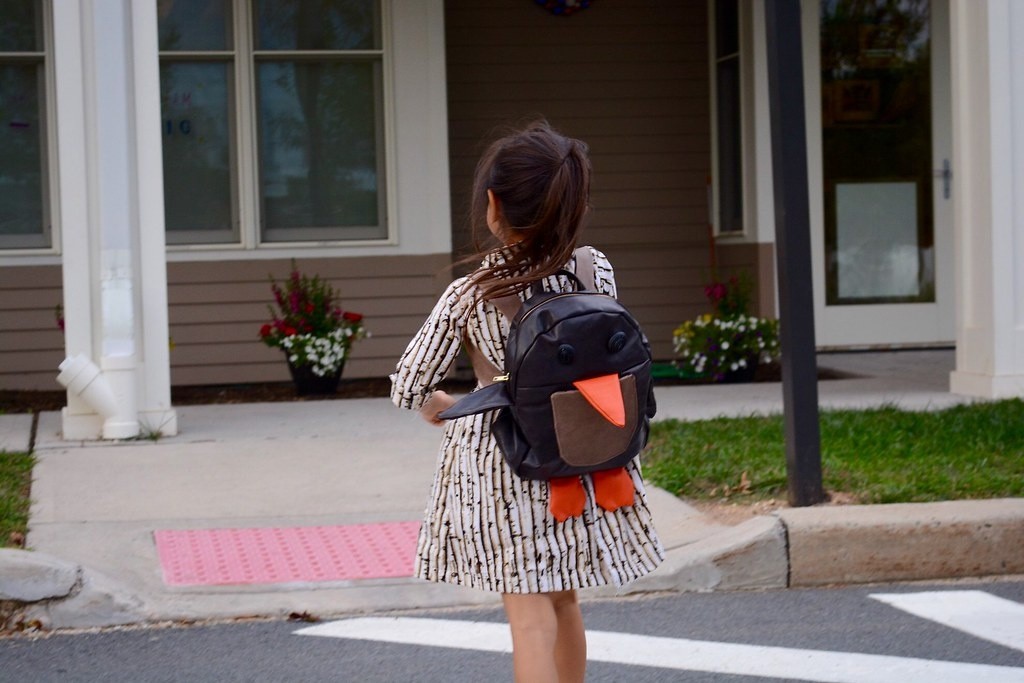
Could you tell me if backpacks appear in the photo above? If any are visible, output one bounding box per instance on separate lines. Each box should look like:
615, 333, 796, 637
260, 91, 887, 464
433, 247, 659, 520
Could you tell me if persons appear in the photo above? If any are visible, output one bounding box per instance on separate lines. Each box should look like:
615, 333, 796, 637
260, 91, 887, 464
387, 117, 665, 683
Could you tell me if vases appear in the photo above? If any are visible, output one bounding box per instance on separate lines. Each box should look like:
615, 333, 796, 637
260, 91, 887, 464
286, 353, 345, 398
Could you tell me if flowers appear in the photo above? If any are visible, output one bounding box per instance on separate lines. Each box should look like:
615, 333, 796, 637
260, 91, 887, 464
669, 273, 781, 385
255, 256, 372, 375
